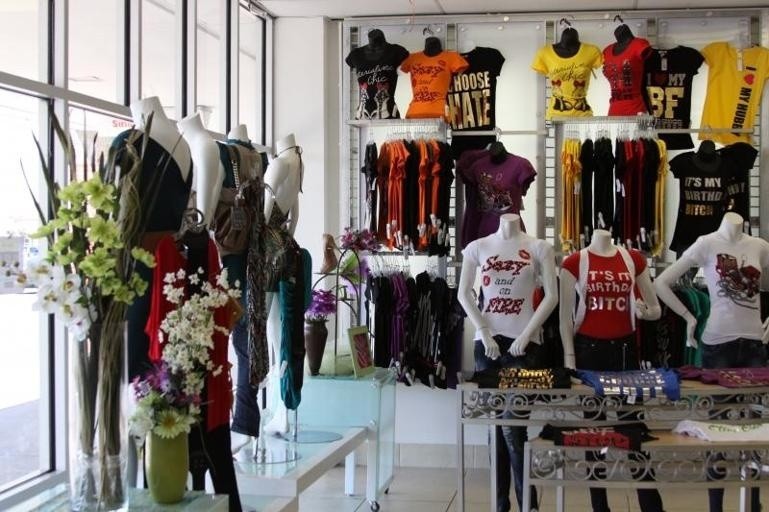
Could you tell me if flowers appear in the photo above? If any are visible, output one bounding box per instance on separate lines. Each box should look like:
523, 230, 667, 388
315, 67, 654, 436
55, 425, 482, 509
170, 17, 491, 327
304, 289, 337, 322
20, 105, 184, 512
128, 266, 242, 460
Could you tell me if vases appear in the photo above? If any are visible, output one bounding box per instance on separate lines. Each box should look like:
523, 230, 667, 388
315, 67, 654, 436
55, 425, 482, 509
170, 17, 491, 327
145, 431, 188, 504
304, 320, 330, 376
71, 450, 129, 512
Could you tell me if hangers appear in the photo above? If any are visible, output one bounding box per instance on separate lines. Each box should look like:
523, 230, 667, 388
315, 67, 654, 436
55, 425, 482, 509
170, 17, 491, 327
369, 250, 448, 282
366, 116, 448, 145
563, 114, 659, 143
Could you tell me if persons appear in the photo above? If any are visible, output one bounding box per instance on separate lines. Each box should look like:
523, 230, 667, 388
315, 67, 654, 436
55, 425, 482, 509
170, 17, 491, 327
458, 213, 559, 511
212, 124, 264, 438
174, 114, 218, 235
651, 212, 767, 511
561, 229, 663, 511
258, 134, 303, 440
108, 98, 192, 488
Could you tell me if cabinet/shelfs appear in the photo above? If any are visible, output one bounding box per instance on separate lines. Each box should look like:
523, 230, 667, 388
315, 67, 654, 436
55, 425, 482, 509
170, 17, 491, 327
456, 369, 769, 512
55, 365, 397, 512
347, 6, 763, 327
